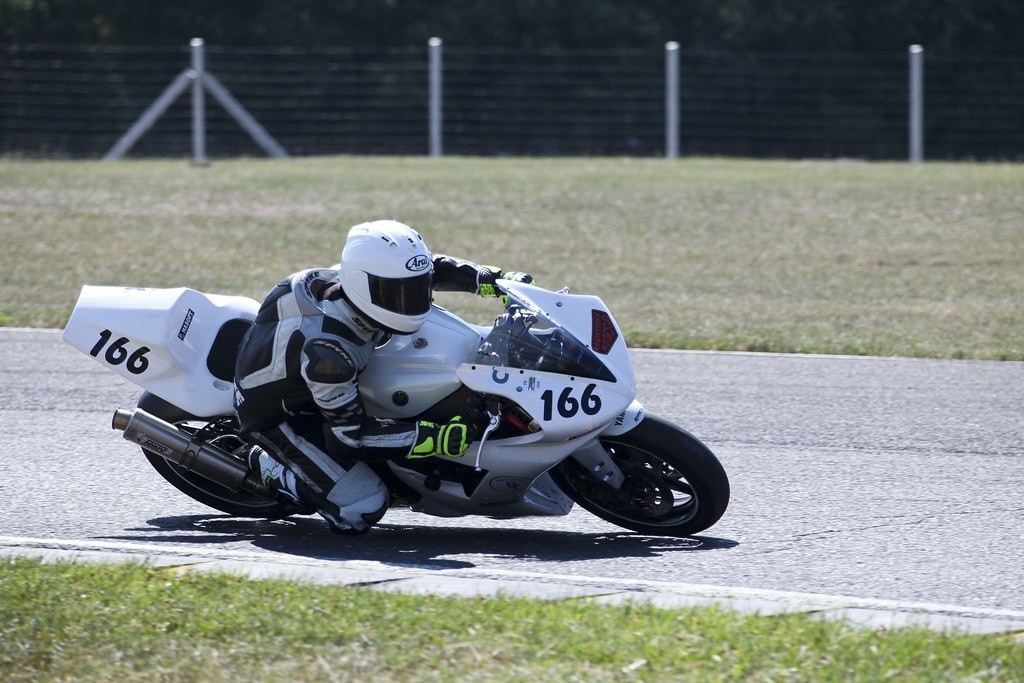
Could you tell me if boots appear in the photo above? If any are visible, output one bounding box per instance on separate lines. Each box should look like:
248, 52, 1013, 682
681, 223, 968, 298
249, 444, 303, 505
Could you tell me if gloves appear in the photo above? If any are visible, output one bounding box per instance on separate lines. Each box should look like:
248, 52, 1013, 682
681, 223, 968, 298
407, 415, 477, 457
479, 265, 535, 308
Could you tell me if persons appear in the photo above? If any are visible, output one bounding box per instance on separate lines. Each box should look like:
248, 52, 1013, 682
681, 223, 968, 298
232, 219, 537, 535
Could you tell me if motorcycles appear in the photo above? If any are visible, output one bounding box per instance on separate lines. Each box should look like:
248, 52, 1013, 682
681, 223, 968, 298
58, 263, 731, 538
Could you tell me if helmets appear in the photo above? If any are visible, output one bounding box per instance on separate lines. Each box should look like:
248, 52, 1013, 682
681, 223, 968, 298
340, 220, 434, 336
